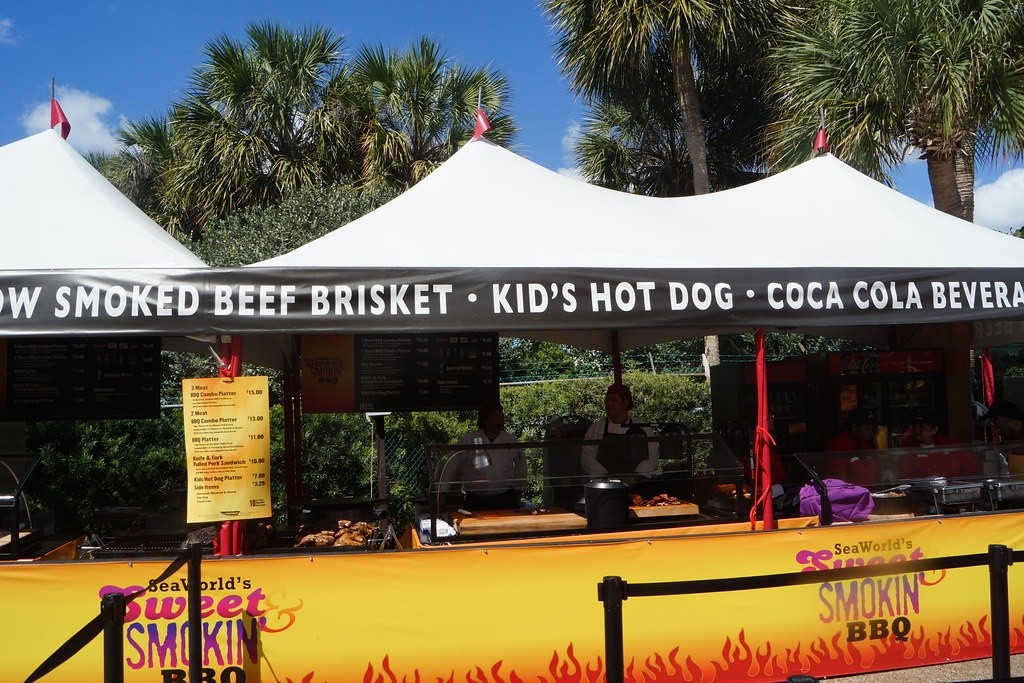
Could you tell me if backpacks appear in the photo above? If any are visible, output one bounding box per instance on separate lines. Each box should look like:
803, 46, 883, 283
799, 479, 876, 522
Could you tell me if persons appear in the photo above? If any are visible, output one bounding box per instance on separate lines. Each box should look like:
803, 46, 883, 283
586, 384, 659, 481
435, 402, 527, 511
898, 406, 980, 479
825, 407, 883, 482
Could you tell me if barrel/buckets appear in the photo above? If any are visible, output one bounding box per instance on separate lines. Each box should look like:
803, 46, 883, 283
584, 478, 630, 532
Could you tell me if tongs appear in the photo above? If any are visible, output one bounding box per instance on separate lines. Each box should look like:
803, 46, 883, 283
873, 484, 912, 493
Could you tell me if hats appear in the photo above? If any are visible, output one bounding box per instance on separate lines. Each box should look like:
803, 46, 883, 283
608, 383, 633, 409
477, 401, 503, 428
904, 412, 936, 425
854, 412, 886, 426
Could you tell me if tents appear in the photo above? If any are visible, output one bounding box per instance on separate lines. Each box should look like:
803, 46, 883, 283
0, 129, 1024, 559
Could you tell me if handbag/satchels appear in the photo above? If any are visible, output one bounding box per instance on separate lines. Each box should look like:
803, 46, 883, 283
773, 485, 794, 516
